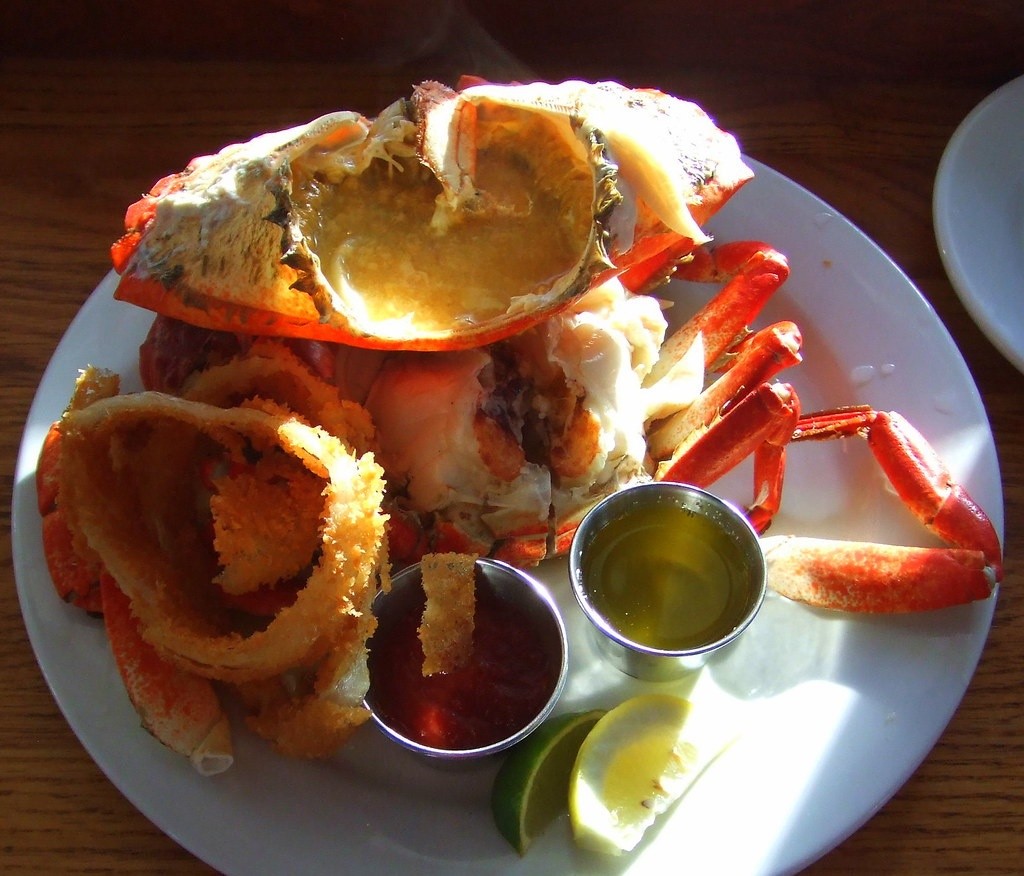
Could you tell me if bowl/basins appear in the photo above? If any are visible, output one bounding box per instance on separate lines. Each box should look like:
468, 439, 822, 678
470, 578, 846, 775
355, 556, 568, 775
567, 481, 769, 683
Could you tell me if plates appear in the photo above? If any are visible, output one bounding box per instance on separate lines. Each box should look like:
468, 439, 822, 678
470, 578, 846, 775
931, 74, 1024, 378
10, 143, 1001, 876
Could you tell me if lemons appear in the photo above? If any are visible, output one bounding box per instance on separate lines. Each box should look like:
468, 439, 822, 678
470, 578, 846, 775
492, 708, 609, 855
570, 694, 751, 855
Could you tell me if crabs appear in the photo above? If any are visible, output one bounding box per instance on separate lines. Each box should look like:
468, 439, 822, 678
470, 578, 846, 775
34, 70, 1005, 780
